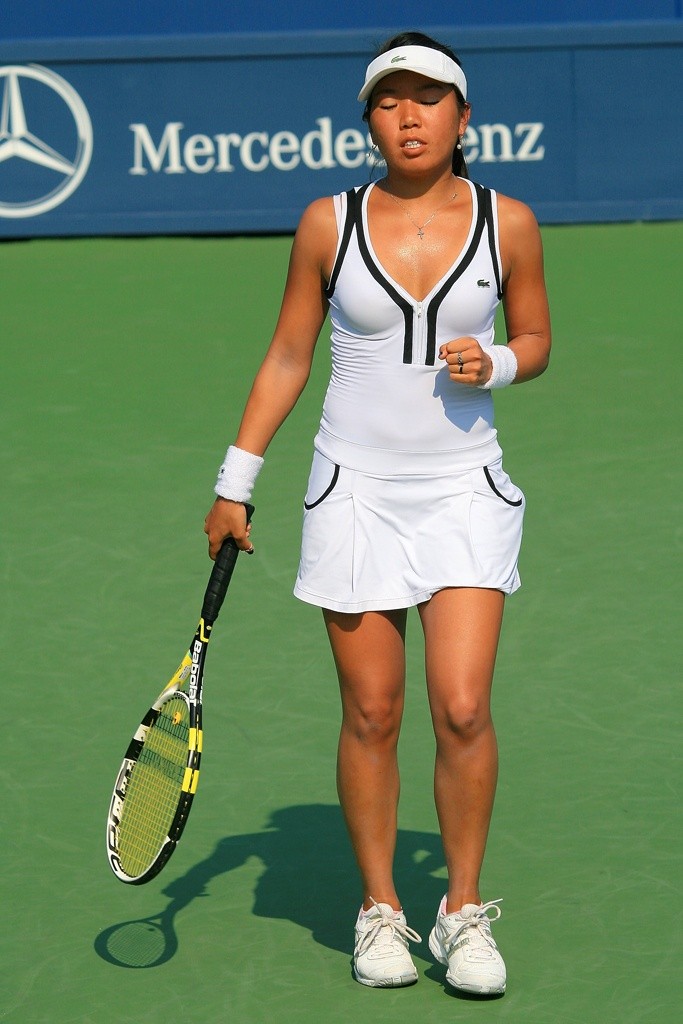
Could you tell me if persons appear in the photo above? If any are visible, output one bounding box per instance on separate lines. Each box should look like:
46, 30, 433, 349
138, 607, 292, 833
204, 32, 550, 993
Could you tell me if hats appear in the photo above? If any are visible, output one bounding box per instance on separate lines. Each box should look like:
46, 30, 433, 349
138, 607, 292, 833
357, 45, 467, 103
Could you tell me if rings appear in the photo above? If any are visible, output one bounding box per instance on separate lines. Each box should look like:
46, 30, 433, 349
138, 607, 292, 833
458, 352, 463, 373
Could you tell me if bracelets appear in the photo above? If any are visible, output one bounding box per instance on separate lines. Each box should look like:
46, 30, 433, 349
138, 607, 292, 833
477, 344, 517, 391
214, 445, 264, 502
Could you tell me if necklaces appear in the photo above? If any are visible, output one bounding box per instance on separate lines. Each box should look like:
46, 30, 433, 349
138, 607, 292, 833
382, 177, 456, 240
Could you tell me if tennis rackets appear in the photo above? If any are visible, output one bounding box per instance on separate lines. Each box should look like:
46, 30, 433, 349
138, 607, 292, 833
104, 502, 257, 886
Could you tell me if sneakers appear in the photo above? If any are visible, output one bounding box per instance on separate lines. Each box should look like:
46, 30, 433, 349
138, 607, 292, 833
353, 895, 419, 989
428, 892, 507, 995
